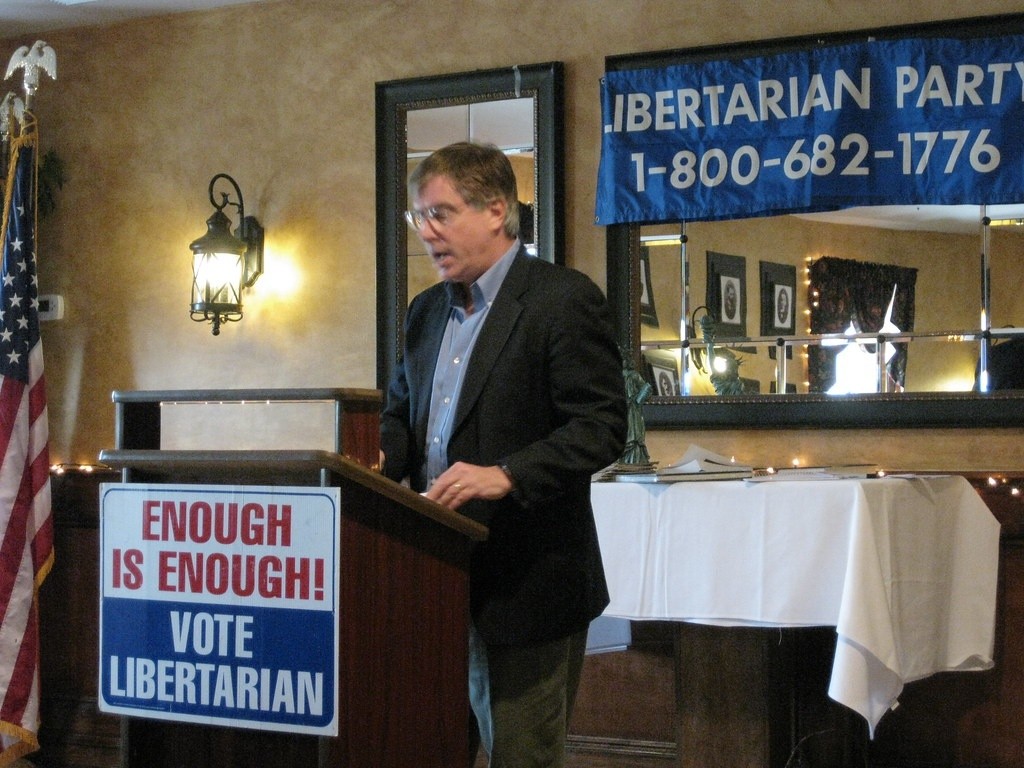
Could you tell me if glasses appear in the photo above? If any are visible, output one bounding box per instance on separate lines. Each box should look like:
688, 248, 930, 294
404, 193, 480, 233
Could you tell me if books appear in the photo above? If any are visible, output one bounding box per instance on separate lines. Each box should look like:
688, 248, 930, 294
777, 463, 878, 474
615, 470, 753, 483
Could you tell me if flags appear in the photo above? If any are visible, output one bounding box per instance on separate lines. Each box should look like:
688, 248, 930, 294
0, 110, 54, 768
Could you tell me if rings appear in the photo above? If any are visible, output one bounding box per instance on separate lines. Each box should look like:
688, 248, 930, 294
453, 483, 462, 490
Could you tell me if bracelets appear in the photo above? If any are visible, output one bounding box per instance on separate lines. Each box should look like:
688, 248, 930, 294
495, 459, 513, 482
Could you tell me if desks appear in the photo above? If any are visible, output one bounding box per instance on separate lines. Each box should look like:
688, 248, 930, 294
591, 475, 1024, 768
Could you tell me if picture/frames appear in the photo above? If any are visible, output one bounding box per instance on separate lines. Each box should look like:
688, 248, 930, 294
758, 260, 800, 336
704, 250, 749, 337
637, 247, 663, 327
643, 349, 678, 396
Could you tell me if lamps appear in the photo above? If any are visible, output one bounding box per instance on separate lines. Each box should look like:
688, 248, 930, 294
684, 307, 741, 374
185, 169, 266, 337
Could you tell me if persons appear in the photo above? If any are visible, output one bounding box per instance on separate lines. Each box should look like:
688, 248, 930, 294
379, 140, 629, 768
973, 338, 1024, 392
621, 357, 651, 464
701, 328, 746, 396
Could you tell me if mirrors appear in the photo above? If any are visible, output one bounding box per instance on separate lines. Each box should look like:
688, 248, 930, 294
603, 9, 1024, 430
376, 60, 564, 390
